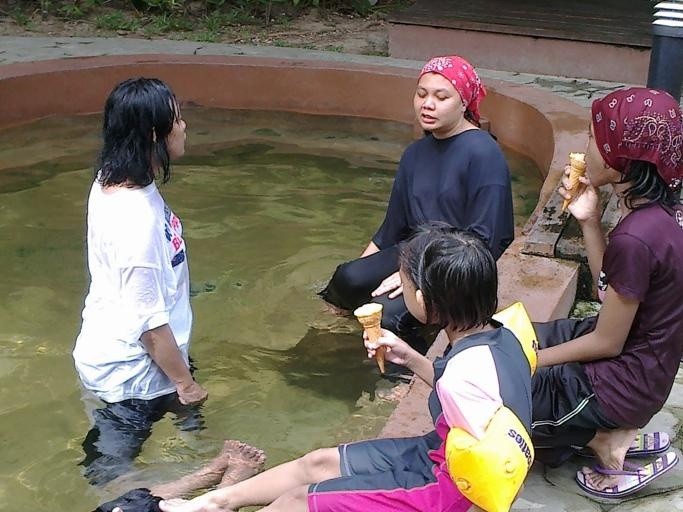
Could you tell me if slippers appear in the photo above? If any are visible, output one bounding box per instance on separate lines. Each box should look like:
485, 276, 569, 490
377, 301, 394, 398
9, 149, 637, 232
575, 452, 679, 499
573, 430, 671, 457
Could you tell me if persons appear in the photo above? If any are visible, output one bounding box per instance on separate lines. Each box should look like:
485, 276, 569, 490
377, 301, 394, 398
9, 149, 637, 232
157, 220, 533, 512
317, 53, 513, 402
509, 85, 683, 499
91, 439, 267, 512
71, 76, 210, 489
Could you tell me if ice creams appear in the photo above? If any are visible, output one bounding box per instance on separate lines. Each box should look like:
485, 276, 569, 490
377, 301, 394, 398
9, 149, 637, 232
354, 301, 386, 375
561, 151, 587, 212
